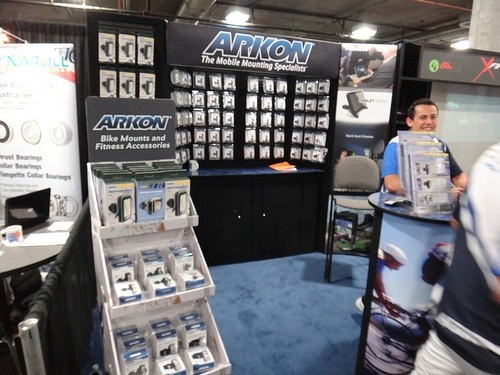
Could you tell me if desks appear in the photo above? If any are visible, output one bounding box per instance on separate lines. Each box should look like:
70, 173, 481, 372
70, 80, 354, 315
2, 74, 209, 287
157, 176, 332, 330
356, 190, 456, 375
0, 216, 75, 349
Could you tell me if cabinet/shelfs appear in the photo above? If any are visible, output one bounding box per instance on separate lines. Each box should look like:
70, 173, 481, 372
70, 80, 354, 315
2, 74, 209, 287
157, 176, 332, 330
193, 178, 321, 267
86, 159, 232, 375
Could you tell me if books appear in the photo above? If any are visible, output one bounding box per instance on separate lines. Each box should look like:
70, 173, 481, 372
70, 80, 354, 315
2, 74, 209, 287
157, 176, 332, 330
269, 161, 297, 171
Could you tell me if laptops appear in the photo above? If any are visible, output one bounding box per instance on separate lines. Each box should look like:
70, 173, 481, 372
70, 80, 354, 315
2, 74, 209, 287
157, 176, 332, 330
0, 187, 51, 236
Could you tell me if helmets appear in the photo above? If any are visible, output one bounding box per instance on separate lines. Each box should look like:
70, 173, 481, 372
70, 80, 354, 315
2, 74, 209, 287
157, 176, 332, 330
383, 243, 405, 268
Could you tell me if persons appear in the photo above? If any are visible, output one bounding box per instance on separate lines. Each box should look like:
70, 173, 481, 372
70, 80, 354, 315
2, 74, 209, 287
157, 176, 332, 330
450, 204, 461, 229
356, 98, 469, 312
409, 141, 500, 375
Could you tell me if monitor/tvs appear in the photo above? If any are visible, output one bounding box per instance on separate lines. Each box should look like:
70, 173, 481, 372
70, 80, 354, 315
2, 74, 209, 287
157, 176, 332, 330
347, 90, 367, 113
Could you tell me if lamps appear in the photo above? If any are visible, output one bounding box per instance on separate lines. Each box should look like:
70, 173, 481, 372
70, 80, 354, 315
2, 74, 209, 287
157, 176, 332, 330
225, 5, 250, 22
350, 21, 378, 40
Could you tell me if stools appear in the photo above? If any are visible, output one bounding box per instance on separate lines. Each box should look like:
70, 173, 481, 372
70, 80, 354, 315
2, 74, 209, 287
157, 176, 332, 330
324, 155, 381, 284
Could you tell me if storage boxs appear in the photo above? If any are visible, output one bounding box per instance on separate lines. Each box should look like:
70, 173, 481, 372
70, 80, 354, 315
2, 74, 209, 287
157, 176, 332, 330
105, 245, 206, 306
90, 161, 191, 224
114, 311, 215, 375
